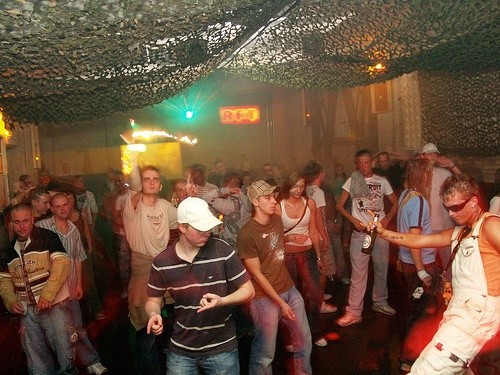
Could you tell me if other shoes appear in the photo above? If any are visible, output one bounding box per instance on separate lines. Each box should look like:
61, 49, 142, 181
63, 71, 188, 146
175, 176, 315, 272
320, 302, 337, 312
316, 338, 326, 347
341, 276, 350, 285
96, 312, 106, 320
323, 292, 331, 301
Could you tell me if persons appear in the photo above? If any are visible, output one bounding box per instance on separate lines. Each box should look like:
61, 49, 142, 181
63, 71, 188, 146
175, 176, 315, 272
0, 143, 500, 375
359, 173, 500, 375
145, 197, 256, 375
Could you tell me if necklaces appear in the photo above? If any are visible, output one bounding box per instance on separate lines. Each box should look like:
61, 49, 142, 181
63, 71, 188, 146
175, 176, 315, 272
286, 198, 302, 206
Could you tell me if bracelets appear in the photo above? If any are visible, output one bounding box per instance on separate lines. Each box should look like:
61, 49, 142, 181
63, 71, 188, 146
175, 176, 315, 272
450, 164, 456, 169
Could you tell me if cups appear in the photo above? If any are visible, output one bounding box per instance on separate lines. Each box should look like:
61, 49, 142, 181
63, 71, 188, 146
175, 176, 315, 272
20, 301, 27, 315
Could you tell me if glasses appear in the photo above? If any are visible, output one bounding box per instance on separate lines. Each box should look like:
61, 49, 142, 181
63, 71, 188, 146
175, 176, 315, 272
442, 193, 476, 213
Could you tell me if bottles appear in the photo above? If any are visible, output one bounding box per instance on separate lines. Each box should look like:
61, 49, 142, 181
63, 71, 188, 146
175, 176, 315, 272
361, 214, 379, 255
410, 282, 425, 304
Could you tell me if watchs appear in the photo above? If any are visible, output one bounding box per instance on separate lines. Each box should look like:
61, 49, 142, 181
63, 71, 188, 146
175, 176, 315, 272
317, 257, 321, 261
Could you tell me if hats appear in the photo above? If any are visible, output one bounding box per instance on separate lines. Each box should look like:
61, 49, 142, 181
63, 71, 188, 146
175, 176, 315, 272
246, 180, 278, 202
422, 142, 439, 152
176, 196, 223, 232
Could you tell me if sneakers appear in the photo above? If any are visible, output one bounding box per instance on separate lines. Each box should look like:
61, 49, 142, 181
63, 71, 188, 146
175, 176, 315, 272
371, 300, 396, 316
336, 313, 360, 327
88, 362, 107, 375
